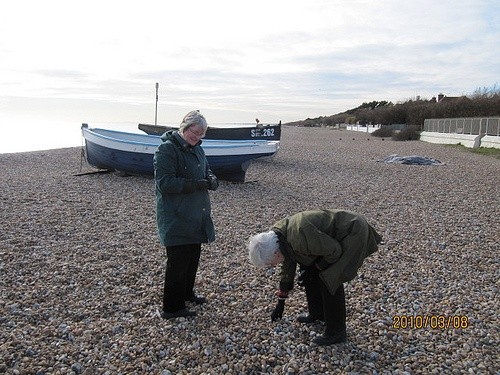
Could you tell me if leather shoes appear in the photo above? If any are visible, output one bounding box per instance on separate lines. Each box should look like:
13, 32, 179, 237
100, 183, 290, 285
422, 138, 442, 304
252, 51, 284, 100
312, 330, 347, 345
160, 307, 197, 320
186, 294, 207, 306
296, 313, 321, 323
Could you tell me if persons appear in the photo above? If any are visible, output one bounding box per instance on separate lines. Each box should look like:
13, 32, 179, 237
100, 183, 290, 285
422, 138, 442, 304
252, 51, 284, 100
155, 110, 217, 318
248, 210, 382, 346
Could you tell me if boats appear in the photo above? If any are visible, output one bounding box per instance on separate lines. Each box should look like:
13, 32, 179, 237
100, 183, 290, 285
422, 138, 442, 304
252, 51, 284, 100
138, 82, 282, 141
81, 122, 281, 182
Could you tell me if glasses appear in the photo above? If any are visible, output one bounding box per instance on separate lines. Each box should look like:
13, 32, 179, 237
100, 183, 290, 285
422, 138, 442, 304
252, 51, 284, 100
188, 127, 205, 138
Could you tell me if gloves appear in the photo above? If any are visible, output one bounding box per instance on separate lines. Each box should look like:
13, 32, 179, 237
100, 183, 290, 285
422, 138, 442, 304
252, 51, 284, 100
192, 178, 209, 191
297, 263, 323, 288
271, 297, 286, 324
207, 174, 219, 191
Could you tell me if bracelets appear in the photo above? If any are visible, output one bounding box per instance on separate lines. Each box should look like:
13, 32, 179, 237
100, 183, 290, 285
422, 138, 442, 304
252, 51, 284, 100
278, 297, 286, 301
